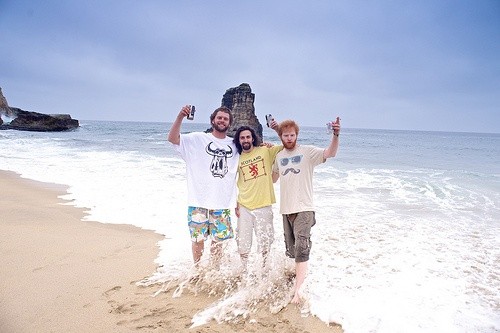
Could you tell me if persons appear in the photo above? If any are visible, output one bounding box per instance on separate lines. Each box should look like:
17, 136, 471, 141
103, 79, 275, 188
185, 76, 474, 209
261, 116, 341, 305
235, 114, 282, 280
168, 105, 275, 278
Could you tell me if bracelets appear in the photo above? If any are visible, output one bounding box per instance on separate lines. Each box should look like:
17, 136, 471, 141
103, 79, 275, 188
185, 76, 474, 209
331, 130, 339, 136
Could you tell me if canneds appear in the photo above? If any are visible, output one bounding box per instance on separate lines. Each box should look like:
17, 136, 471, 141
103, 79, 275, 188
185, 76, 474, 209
187, 105, 195, 120
325, 120, 335, 135
265, 113, 273, 127
